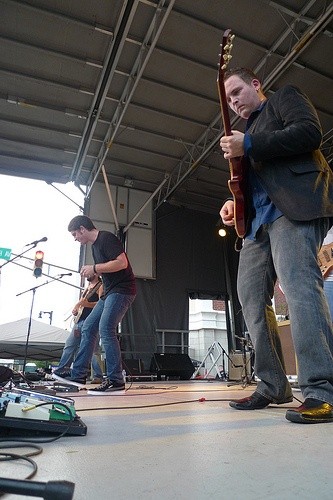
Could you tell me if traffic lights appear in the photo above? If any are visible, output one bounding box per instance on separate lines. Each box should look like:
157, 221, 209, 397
33, 250, 44, 277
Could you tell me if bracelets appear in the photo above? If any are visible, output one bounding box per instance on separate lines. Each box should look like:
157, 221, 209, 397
93, 263, 97, 274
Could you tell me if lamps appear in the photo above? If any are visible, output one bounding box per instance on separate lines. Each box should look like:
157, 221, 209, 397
215, 219, 232, 238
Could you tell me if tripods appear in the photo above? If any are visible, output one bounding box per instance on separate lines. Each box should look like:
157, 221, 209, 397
222, 241, 258, 389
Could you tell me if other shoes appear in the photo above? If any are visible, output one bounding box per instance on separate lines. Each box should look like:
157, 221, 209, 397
61, 379, 86, 387
285, 399, 333, 423
229, 391, 294, 410
92, 378, 103, 384
89, 379, 126, 394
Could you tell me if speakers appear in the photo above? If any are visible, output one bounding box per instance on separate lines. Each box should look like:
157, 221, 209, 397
277, 319, 298, 380
149, 352, 196, 381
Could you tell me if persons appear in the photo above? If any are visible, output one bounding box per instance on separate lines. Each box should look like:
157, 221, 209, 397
53, 216, 138, 393
45, 272, 105, 384
220, 65, 333, 423
322, 226, 333, 325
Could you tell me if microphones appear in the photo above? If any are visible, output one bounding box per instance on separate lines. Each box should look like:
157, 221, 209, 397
26, 237, 47, 246
60, 272, 72, 276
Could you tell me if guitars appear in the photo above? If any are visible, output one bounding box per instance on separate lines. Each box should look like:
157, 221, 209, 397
216, 28, 252, 238
74, 288, 92, 324
278, 241, 333, 301
